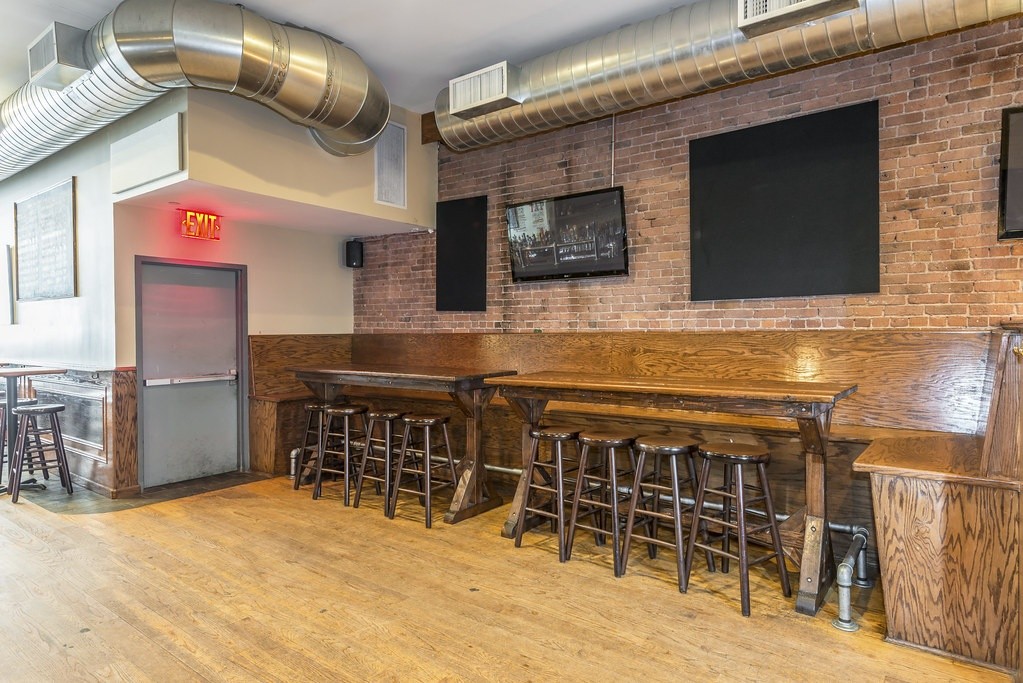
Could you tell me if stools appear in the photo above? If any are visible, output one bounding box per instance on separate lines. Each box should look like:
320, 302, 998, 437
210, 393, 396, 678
515, 425, 791, 616
293, 401, 458, 528
0, 397, 74, 504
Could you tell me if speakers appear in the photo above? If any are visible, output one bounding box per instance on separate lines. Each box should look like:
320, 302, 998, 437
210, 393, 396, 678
346, 241, 364, 268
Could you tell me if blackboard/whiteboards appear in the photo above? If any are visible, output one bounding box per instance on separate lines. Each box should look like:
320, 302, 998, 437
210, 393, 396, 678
689, 100, 880, 301
436, 195, 487, 311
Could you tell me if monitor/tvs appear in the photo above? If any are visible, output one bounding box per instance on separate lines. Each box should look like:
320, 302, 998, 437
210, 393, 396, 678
506, 186, 630, 283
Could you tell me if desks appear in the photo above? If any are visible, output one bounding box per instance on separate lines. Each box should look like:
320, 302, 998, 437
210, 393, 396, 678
285, 363, 518, 524
0, 366, 67, 494
484, 371, 859, 617
